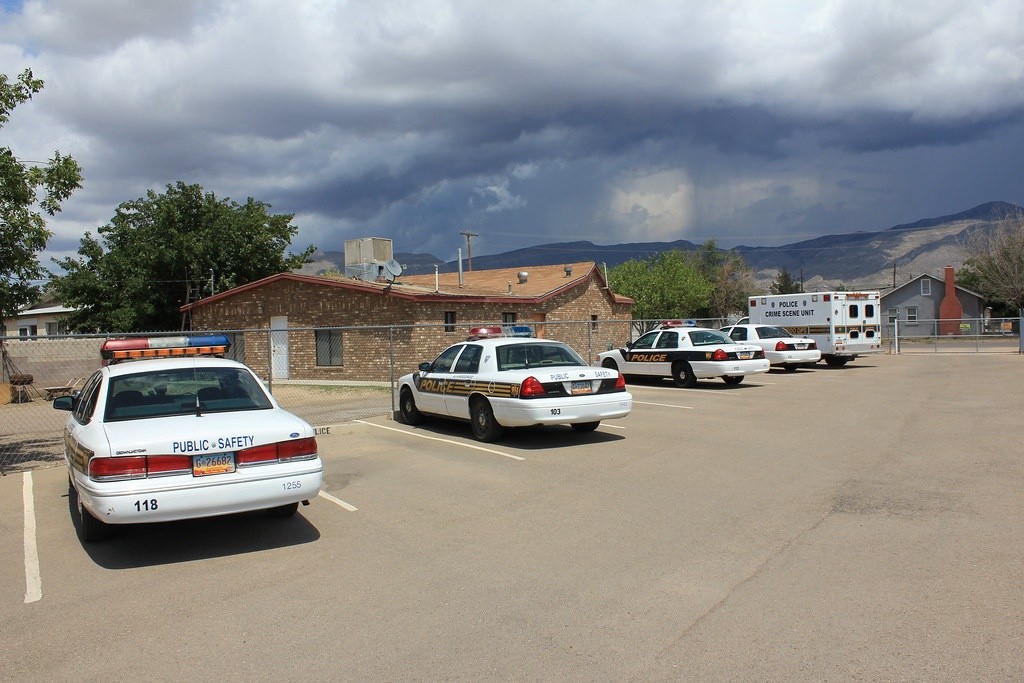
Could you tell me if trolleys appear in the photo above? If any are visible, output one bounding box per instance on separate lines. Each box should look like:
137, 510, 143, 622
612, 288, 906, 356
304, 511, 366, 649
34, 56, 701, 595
44, 377, 86, 401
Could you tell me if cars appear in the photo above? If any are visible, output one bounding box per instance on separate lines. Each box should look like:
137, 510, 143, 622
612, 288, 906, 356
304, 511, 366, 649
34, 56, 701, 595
396, 326, 633, 443
717, 324, 822, 373
53, 334, 325, 544
594, 320, 771, 388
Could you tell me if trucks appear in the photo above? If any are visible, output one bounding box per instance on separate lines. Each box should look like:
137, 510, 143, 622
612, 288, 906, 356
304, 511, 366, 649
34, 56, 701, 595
734, 291, 885, 368
